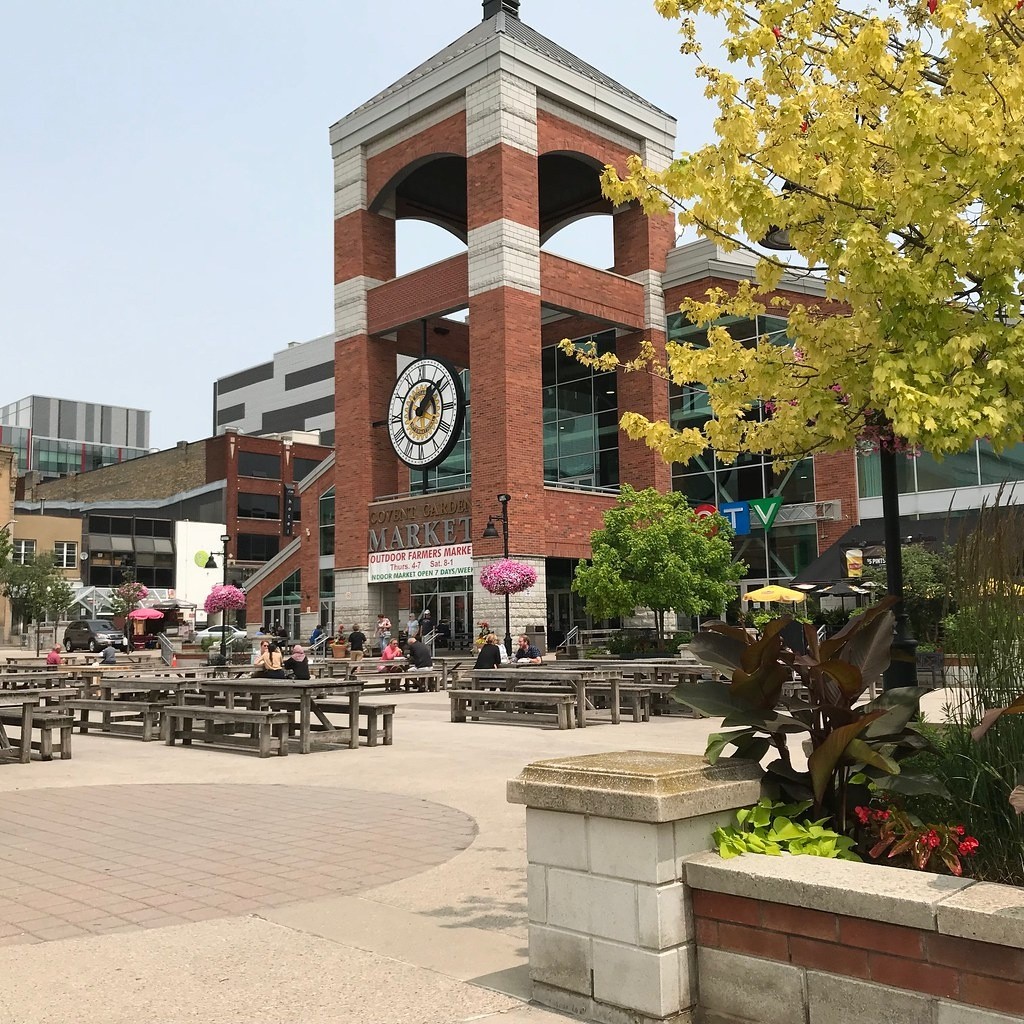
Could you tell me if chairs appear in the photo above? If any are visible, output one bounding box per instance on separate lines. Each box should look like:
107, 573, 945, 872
0, 653, 715, 760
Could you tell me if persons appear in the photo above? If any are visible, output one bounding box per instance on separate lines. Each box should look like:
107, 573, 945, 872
276, 626, 290, 649
284, 644, 310, 680
47, 643, 65, 664
348, 623, 368, 671
436, 619, 450, 648
374, 614, 391, 655
403, 613, 420, 649
253, 640, 270, 662
419, 610, 432, 656
268, 627, 277, 636
494, 635, 508, 664
101, 641, 116, 664
510, 634, 542, 664
407, 637, 435, 693
310, 625, 323, 655
256, 627, 265, 636
473, 633, 501, 669
376, 638, 404, 692
254, 643, 284, 679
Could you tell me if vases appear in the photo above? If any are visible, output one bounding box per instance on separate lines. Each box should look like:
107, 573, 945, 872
330, 644, 349, 658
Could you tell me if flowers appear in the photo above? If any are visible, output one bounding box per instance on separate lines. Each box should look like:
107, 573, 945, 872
481, 559, 537, 596
204, 584, 246, 613
329, 638, 350, 648
118, 583, 149, 604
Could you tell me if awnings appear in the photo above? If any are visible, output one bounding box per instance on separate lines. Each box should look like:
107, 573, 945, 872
787, 515, 960, 598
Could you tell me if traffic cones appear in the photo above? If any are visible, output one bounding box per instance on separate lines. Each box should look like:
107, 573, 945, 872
171, 655, 178, 668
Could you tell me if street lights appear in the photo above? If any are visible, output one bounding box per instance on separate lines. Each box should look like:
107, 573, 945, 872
204, 535, 232, 666
481, 493, 512, 656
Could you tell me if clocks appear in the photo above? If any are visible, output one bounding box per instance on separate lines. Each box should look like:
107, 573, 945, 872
389, 357, 467, 471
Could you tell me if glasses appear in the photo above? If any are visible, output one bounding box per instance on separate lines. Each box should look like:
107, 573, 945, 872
392, 642, 398, 645
261, 644, 269, 647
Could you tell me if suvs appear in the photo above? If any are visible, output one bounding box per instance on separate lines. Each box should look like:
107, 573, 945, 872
62, 619, 129, 653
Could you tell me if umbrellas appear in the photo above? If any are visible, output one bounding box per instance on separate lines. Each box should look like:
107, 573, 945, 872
950, 576, 1024, 597
742, 585, 808, 604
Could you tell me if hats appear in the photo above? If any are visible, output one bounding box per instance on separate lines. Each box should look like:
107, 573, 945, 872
423, 610, 431, 614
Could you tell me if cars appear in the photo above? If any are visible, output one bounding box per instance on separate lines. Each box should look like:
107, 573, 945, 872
194, 624, 247, 640
610, 627, 691, 650
